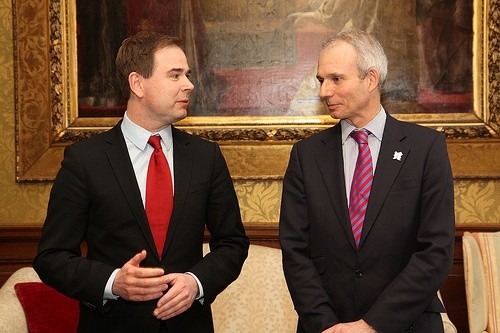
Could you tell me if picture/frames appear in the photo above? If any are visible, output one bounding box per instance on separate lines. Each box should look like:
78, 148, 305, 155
12, 0, 500, 184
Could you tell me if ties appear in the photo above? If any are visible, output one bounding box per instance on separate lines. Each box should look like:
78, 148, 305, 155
348, 129, 374, 249
146, 136, 174, 262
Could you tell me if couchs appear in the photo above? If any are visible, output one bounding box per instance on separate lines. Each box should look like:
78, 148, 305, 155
0, 241, 458, 333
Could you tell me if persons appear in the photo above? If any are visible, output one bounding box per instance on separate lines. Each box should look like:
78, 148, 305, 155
277, 29, 456, 333
32, 29, 251, 333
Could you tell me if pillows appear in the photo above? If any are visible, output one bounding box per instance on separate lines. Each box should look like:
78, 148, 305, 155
14, 282, 80, 333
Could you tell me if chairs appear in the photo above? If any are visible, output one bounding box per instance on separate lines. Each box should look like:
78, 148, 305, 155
462, 231, 500, 333
